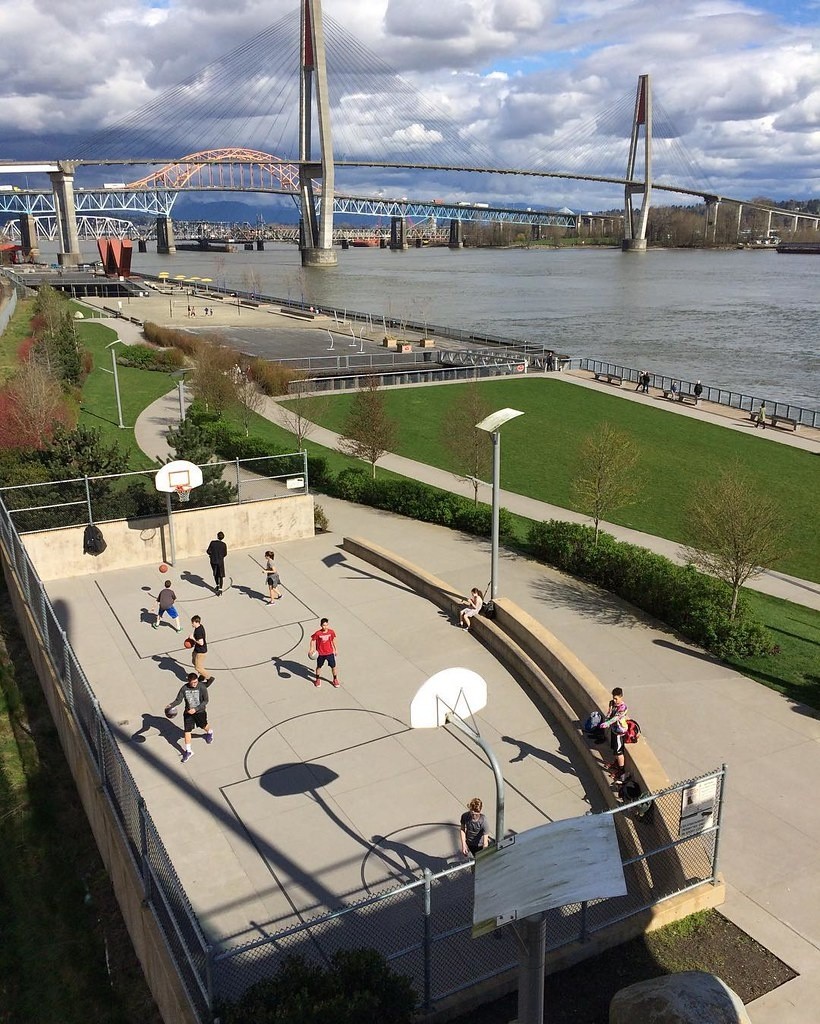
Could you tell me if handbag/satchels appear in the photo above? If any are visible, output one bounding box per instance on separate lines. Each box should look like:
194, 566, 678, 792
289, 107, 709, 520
634, 793, 652, 813
585, 711, 603, 731
622, 720, 641, 742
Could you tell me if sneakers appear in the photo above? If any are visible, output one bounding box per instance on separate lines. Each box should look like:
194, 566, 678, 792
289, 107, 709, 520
315, 679, 321, 687
205, 731, 213, 743
333, 679, 340, 687
181, 751, 192, 762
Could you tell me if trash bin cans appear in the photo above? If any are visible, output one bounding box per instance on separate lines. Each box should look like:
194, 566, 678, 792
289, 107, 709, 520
140, 292, 143, 298
145, 291, 149, 297
390, 320, 396, 328
118, 302, 122, 309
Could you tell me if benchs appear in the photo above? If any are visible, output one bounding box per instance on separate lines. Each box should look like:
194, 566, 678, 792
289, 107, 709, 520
772, 415, 802, 432
750, 411, 772, 421
593, 372, 628, 386
663, 390, 702, 407
344, 536, 714, 905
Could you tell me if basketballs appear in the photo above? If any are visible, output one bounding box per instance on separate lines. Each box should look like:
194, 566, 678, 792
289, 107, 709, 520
159, 564, 168, 573
166, 707, 177, 719
184, 638, 195, 649
308, 650, 319, 661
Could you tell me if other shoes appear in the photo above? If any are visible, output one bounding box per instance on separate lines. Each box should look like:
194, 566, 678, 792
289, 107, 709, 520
277, 594, 283, 599
152, 623, 158, 628
216, 590, 222, 597
215, 586, 219, 591
604, 762, 617, 769
462, 627, 473, 631
177, 628, 183, 633
198, 675, 205, 682
611, 770, 626, 779
266, 601, 276, 606
455, 623, 464, 628
205, 676, 215, 687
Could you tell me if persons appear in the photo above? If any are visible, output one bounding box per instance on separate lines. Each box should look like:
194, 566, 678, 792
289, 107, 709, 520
455, 587, 484, 632
250, 292, 254, 300
210, 308, 213, 315
166, 673, 214, 763
232, 363, 253, 390
600, 687, 630, 779
670, 382, 677, 401
694, 380, 702, 406
634, 371, 649, 394
310, 305, 322, 315
309, 618, 340, 688
152, 580, 184, 634
545, 352, 553, 372
204, 308, 208, 317
755, 404, 765, 430
460, 798, 489, 874
262, 551, 284, 607
206, 532, 227, 597
188, 305, 196, 317
188, 615, 215, 688
